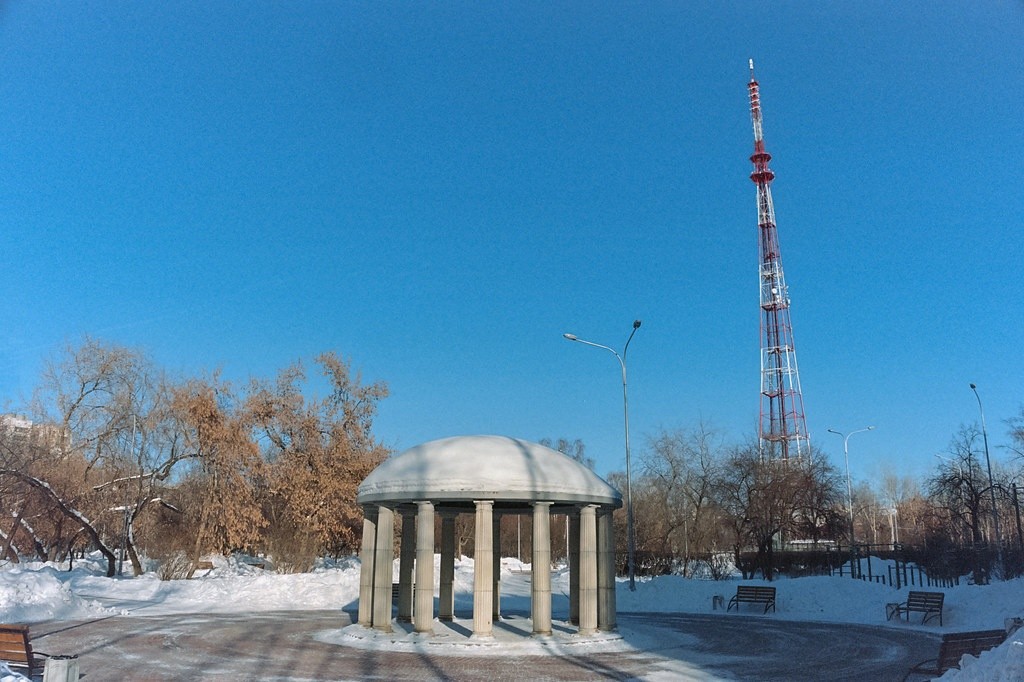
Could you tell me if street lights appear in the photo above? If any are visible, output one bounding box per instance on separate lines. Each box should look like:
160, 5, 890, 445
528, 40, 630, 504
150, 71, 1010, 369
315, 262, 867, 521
969, 382, 1005, 583
826, 425, 877, 580
563, 320, 645, 591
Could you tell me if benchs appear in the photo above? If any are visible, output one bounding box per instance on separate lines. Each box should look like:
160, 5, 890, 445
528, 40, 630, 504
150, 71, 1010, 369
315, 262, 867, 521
0, 624, 78, 680
904, 630, 1006, 682
887, 591, 945, 627
727, 585, 776, 615
